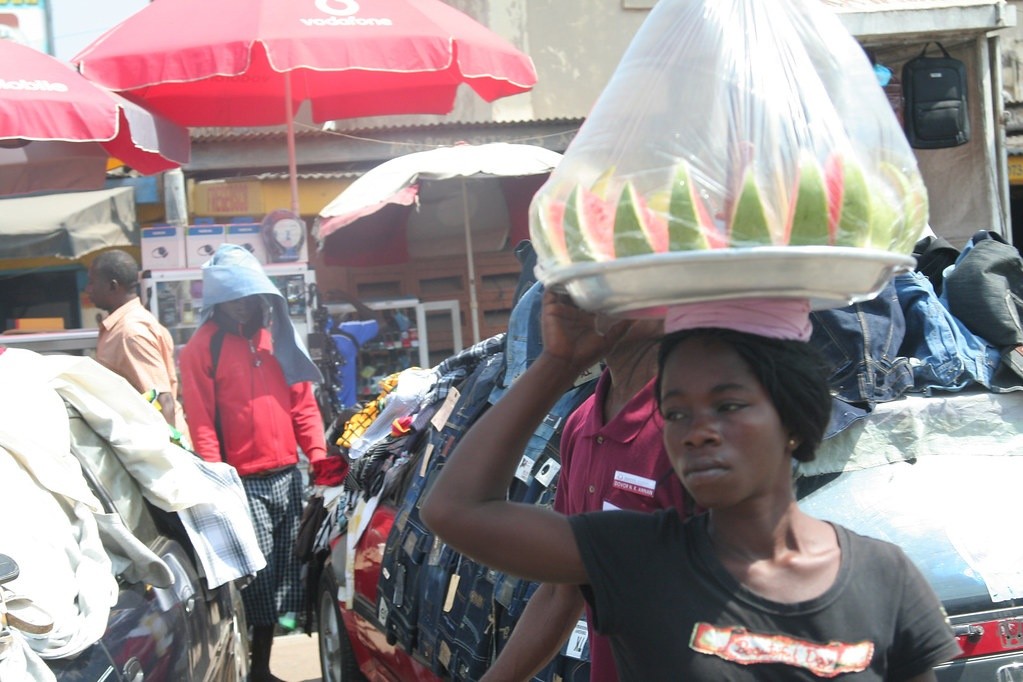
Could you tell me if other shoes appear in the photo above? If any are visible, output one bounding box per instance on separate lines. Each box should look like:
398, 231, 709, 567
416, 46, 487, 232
247, 670, 284, 682
1, 549, 54, 635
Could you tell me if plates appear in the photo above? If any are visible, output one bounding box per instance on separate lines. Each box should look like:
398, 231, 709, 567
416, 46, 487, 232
544, 245, 918, 319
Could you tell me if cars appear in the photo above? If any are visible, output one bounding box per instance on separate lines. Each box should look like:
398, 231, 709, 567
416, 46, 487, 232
312, 389, 1023, 682
0, 353, 261, 682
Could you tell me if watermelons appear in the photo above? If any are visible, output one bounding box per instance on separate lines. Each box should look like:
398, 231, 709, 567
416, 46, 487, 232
530, 146, 929, 266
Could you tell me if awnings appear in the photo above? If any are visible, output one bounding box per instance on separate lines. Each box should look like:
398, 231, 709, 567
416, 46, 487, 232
0, 186, 141, 260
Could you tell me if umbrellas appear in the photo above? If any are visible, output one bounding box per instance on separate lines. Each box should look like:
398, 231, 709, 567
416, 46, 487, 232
318, 141, 564, 345
0, 36, 190, 197
68, 0, 538, 216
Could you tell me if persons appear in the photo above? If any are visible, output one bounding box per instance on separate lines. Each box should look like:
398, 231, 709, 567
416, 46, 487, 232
478, 319, 705, 682
85, 249, 179, 429
417, 289, 965, 682
179, 244, 327, 682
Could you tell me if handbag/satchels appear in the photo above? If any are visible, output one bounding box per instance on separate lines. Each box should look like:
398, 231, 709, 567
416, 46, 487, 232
901, 40, 970, 150
